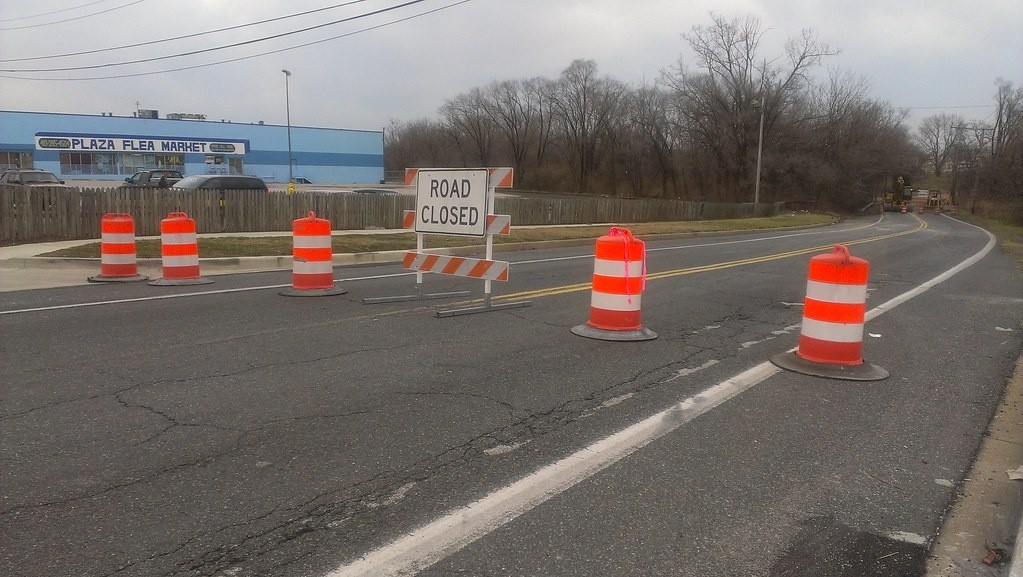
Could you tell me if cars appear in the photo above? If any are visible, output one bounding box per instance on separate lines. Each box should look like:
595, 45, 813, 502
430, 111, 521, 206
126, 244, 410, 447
168, 174, 269, 210
0, 169, 73, 210
123, 169, 184, 187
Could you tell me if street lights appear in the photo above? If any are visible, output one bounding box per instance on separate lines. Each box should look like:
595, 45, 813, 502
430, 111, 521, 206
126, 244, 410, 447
282, 69, 292, 183
752, 97, 765, 207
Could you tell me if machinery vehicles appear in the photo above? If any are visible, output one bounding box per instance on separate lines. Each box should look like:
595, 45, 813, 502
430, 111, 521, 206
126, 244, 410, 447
882, 177, 914, 212
923, 189, 947, 213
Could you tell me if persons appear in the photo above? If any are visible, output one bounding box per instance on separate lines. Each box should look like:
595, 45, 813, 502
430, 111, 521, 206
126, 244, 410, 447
157, 176, 169, 189
